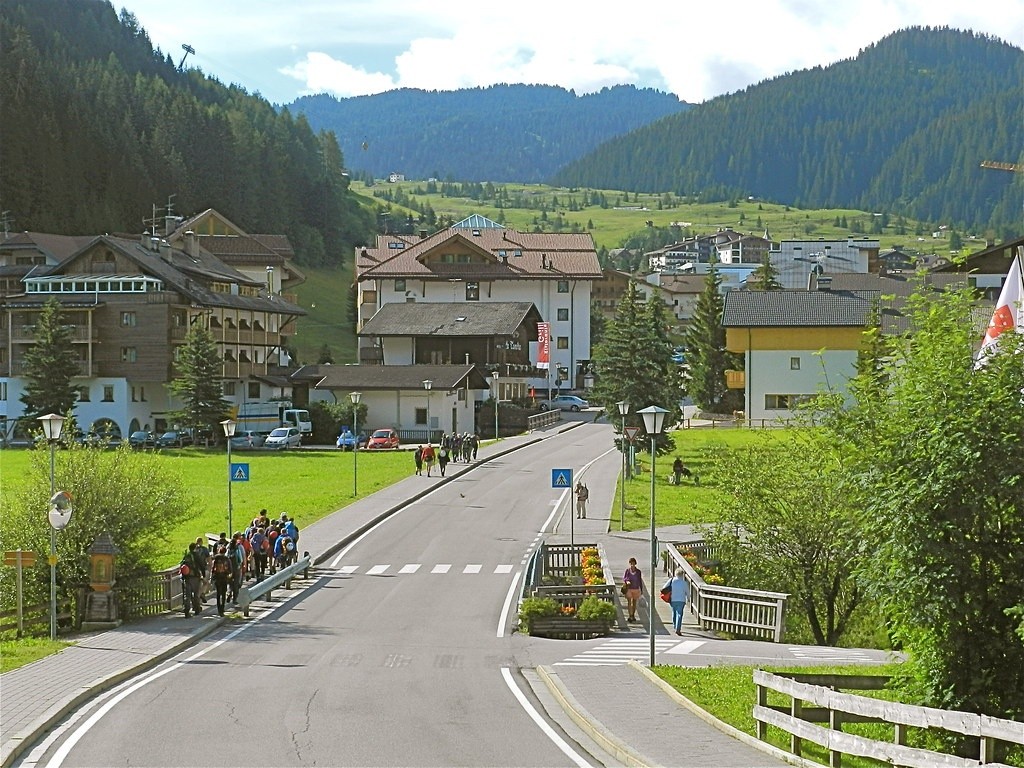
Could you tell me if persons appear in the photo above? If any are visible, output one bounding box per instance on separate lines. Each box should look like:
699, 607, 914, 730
663, 569, 689, 636
623, 558, 643, 622
415, 443, 436, 477
440, 432, 480, 463
574, 483, 587, 519
437, 446, 448, 477
673, 457, 683, 484
180, 510, 299, 619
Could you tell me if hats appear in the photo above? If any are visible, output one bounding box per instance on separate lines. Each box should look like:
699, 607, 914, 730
280, 512, 288, 518
576, 481, 581, 485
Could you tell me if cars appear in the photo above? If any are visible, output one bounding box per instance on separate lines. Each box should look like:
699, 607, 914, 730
366, 428, 399, 449
336, 433, 360, 450
81, 431, 114, 443
231, 430, 264, 450
538, 395, 589, 412
264, 427, 302, 450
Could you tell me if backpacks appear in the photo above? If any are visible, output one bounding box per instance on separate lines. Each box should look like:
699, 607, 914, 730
280, 536, 297, 558
225, 543, 241, 571
213, 557, 230, 575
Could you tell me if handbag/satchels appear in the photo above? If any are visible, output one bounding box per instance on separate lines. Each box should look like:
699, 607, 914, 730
660, 577, 673, 603
424, 456, 433, 462
620, 580, 632, 594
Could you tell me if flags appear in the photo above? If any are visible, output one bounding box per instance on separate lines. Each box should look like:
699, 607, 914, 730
974, 256, 1024, 372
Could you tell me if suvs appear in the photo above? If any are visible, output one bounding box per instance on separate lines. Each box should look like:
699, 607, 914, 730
194, 429, 216, 447
128, 429, 158, 447
155, 430, 193, 448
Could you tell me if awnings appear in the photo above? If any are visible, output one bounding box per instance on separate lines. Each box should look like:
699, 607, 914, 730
255, 375, 292, 387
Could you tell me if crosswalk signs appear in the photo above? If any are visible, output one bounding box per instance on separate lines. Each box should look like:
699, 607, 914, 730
551, 469, 571, 487
230, 463, 249, 481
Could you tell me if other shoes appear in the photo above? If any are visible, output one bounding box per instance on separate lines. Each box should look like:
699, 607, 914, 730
577, 517, 580, 519
628, 616, 636, 623
195, 607, 202, 615
582, 517, 586, 519
200, 593, 207, 603
673, 626, 676, 629
676, 630, 681, 636
185, 612, 193, 618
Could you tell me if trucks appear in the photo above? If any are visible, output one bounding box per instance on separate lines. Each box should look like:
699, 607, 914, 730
236, 400, 313, 440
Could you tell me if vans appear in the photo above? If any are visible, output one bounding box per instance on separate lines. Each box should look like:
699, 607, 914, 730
346, 430, 367, 449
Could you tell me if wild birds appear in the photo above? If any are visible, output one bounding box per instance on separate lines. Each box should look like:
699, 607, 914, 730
460, 494, 466, 498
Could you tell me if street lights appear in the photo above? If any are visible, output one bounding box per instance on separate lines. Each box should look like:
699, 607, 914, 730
219, 419, 238, 539
491, 371, 500, 440
36, 412, 68, 641
422, 379, 433, 442
615, 400, 632, 530
555, 361, 561, 395
349, 390, 362, 496
635, 405, 671, 667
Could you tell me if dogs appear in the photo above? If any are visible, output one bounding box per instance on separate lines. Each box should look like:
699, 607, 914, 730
694, 477, 699, 486
682, 468, 691, 478
